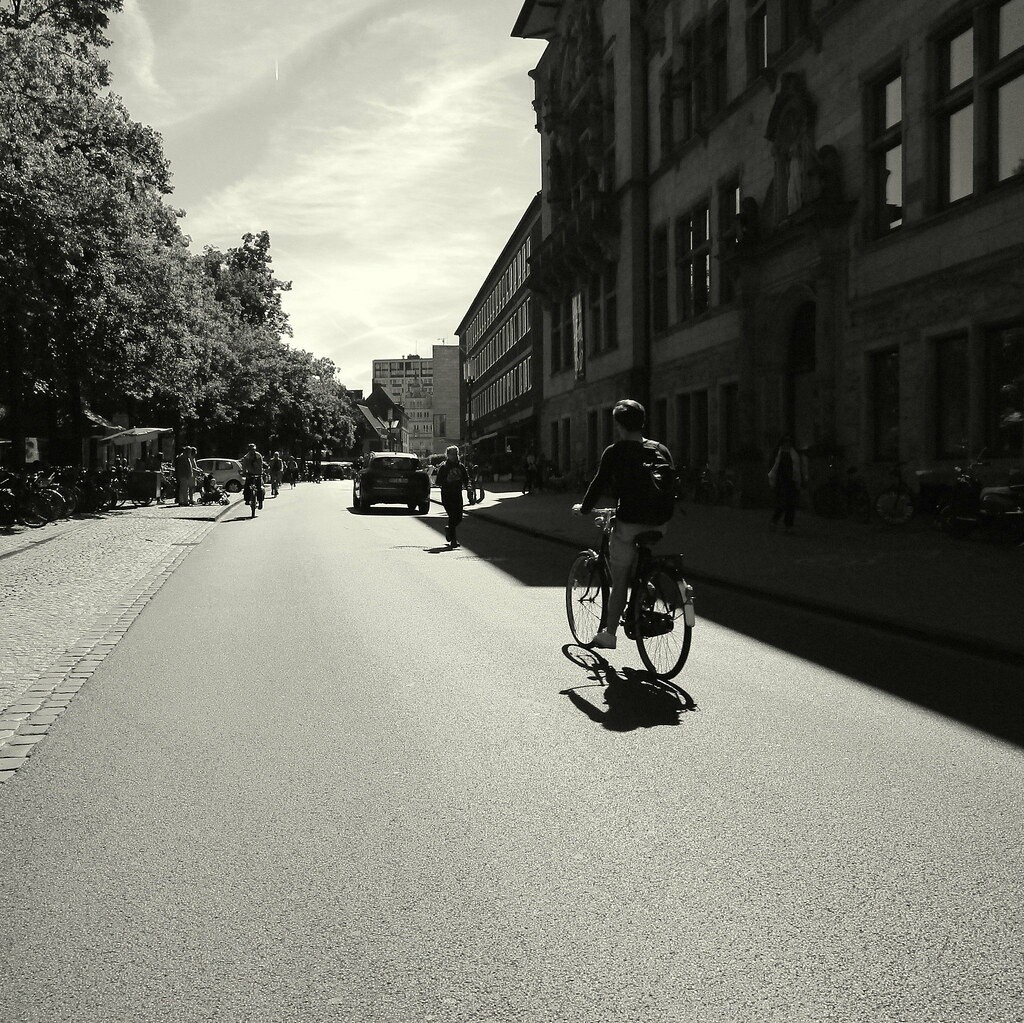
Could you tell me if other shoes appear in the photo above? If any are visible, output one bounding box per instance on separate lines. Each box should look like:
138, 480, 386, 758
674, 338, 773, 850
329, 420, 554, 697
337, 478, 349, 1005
594, 632, 615, 648
445, 524, 450, 540
642, 581, 655, 595
451, 543, 460, 548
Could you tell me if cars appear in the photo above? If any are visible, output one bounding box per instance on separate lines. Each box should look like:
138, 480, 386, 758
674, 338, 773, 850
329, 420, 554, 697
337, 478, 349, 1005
194, 458, 246, 492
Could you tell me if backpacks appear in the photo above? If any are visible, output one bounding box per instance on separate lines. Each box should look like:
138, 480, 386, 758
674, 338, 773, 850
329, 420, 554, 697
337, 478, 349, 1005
615, 440, 675, 500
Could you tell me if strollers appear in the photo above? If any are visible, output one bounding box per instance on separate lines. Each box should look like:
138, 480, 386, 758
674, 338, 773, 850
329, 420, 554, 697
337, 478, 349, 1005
192, 468, 230, 507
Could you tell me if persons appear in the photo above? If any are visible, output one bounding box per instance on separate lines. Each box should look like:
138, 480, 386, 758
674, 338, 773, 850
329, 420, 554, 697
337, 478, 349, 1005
170, 442, 321, 509
521, 446, 552, 496
571, 399, 675, 648
434, 445, 485, 548
768, 433, 806, 535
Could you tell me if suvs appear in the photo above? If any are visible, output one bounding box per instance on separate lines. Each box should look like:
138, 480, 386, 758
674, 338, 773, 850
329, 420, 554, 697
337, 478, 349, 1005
355, 451, 430, 515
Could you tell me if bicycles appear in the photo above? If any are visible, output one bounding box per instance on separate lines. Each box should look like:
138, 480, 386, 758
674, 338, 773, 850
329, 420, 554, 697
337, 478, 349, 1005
518, 445, 1024, 547
0, 460, 177, 529
271, 471, 280, 496
289, 466, 296, 486
239, 472, 263, 517
564, 507, 695, 682
